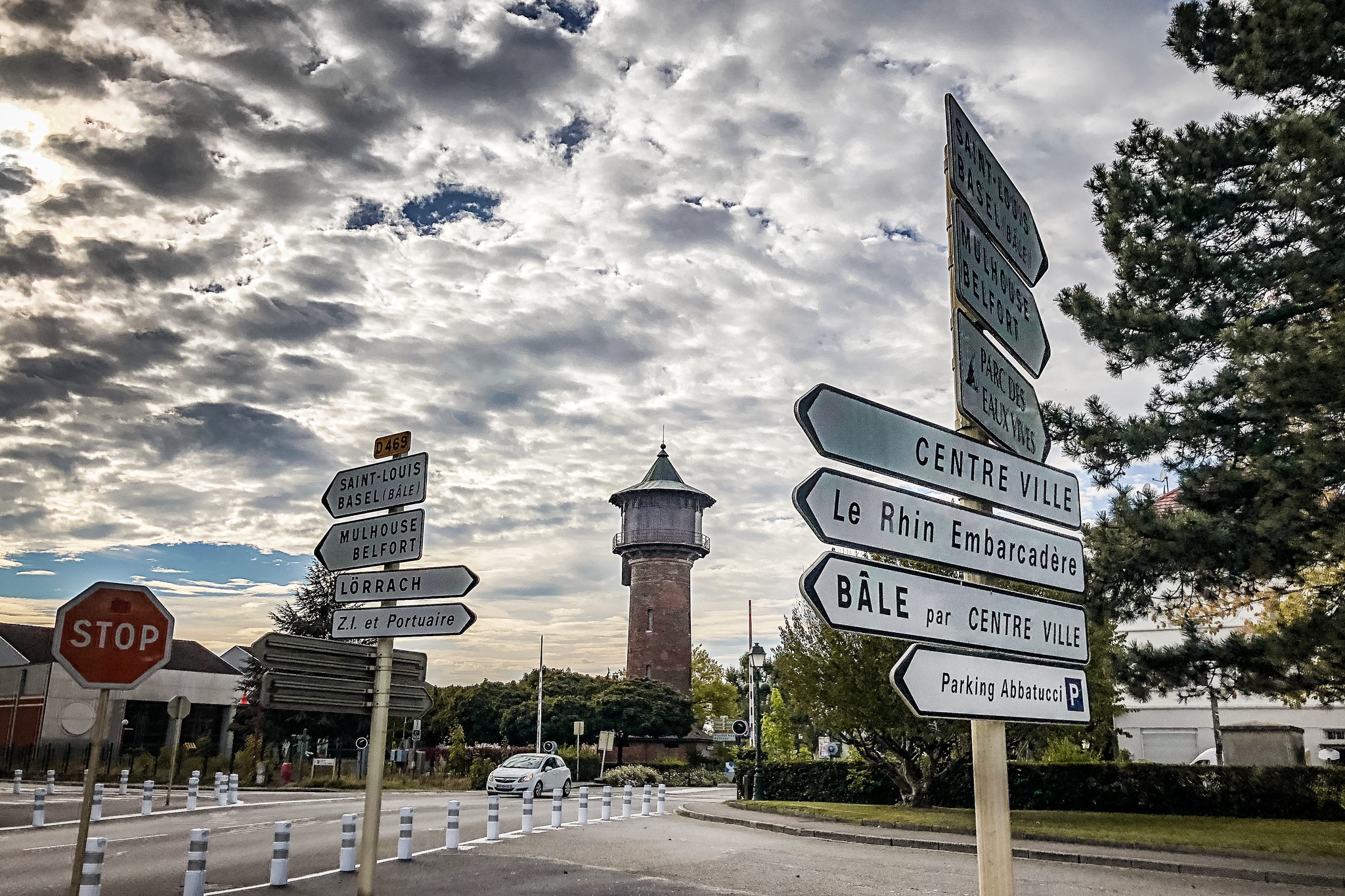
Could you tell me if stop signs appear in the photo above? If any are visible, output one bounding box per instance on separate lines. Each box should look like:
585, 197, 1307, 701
51, 581, 175, 692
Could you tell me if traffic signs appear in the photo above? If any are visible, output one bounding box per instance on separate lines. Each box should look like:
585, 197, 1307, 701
791, 87, 1093, 729
316, 429, 479, 638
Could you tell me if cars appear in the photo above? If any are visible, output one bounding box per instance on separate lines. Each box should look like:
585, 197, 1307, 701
486, 753, 572, 798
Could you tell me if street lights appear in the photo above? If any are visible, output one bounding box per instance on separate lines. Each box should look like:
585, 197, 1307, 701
748, 642, 767, 801
